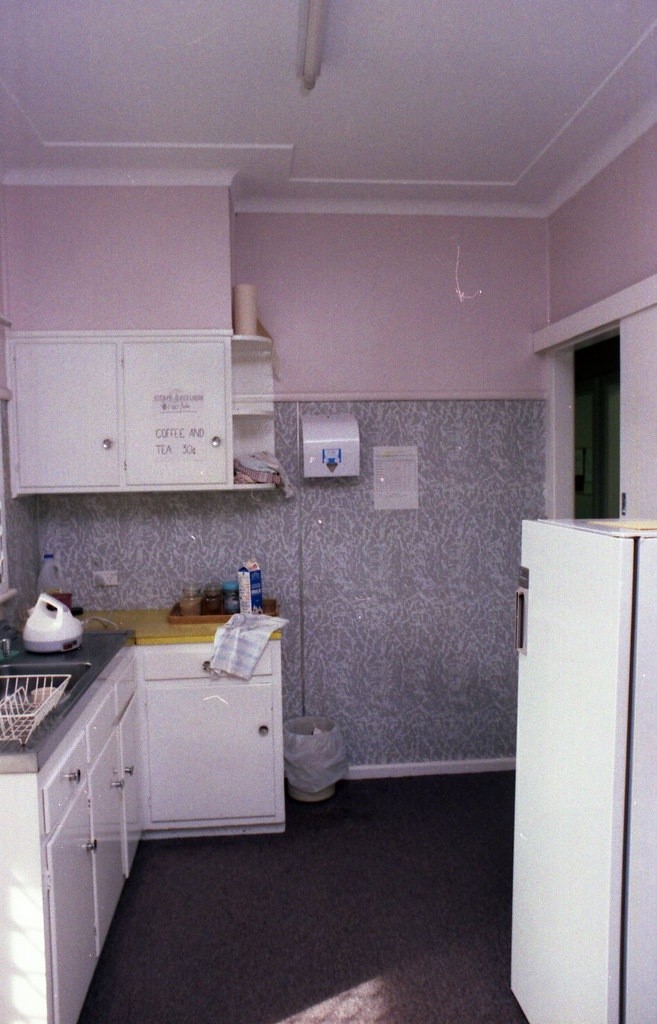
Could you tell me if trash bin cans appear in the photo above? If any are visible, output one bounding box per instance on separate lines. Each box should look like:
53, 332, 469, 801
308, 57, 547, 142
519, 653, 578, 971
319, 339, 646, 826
283, 716, 338, 802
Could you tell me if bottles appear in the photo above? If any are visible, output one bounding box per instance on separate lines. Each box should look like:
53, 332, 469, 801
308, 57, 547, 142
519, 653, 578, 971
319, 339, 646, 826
201, 584, 223, 614
179, 584, 202, 617
36, 554, 62, 597
221, 582, 240, 614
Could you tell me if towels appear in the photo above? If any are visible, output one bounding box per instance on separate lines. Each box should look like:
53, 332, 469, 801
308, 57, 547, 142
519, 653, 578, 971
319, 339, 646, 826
209, 612, 290, 682
229, 451, 296, 500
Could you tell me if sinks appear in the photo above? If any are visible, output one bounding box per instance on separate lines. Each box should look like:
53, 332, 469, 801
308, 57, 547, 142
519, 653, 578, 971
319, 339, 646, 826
0, 662, 93, 705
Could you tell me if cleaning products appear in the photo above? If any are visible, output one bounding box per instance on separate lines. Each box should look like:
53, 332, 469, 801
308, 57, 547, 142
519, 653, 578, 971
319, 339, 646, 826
35, 553, 65, 598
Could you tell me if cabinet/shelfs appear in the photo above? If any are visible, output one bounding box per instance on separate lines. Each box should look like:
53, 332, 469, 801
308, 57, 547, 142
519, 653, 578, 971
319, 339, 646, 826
0, 640, 287, 1024
6, 329, 277, 499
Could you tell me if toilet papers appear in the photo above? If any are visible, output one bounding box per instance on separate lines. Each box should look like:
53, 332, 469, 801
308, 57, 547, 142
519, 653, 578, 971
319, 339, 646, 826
235, 282, 284, 380
